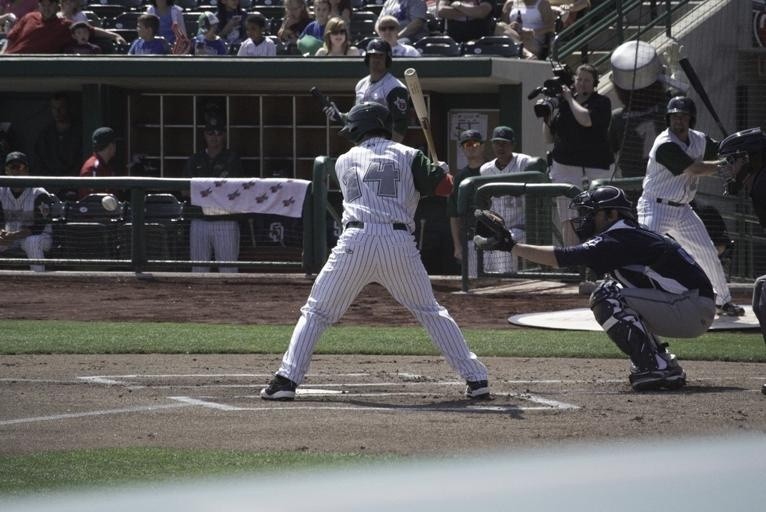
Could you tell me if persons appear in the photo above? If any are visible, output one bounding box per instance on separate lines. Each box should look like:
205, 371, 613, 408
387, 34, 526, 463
718, 126, 766, 395
447, 125, 545, 272
321, 38, 409, 146
182, 118, 244, 273
259, 100, 492, 402
0, 0, 614, 56
472, 182, 718, 389
33, 91, 83, 187
545, 62, 615, 245
0, 152, 54, 271
78, 127, 122, 259
633, 96, 746, 317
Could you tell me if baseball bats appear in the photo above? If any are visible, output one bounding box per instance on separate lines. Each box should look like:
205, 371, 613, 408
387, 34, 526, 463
679, 57, 728, 139
311, 86, 345, 127
404, 67, 439, 165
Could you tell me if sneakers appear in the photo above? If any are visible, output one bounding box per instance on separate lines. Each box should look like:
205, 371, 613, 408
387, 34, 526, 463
629, 355, 686, 392
716, 304, 745, 316
260, 375, 296, 401
464, 379, 489, 398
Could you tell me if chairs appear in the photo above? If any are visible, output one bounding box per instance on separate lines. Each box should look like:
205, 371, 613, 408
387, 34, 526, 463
87, 0, 591, 57
0, 193, 299, 271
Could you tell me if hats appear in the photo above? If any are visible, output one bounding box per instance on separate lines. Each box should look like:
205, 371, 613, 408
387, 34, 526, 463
197, 12, 219, 35
204, 117, 224, 131
338, 102, 392, 150
365, 39, 392, 67
488, 126, 517, 145
93, 127, 124, 144
459, 130, 483, 146
5, 151, 33, 172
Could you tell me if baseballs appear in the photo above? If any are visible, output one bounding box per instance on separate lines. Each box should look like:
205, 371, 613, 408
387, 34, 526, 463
101, 194, 118, 212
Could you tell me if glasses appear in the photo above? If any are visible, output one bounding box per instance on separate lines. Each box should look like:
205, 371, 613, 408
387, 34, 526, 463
207, 129, 221, 136
381, 25, 395, 31
332, 29, 346, 35
462, 142, 481, 149
725, 153, 747, 165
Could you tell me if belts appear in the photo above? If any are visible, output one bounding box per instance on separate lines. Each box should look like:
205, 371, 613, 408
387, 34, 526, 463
200, 217, 237, 222
657, 197, 684, 207
698, 289, 715, 300
346, 221, 409, 231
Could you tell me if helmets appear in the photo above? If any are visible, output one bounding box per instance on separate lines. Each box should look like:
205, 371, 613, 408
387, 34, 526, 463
568, 185, 634, 241
666, 96, 697, 130
717, 127, 765, 199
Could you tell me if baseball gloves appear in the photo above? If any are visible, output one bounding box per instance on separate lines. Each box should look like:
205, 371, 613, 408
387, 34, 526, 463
474, 209, 515, 253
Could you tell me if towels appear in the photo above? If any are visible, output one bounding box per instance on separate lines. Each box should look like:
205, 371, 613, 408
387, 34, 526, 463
190, 176, 312, 218
